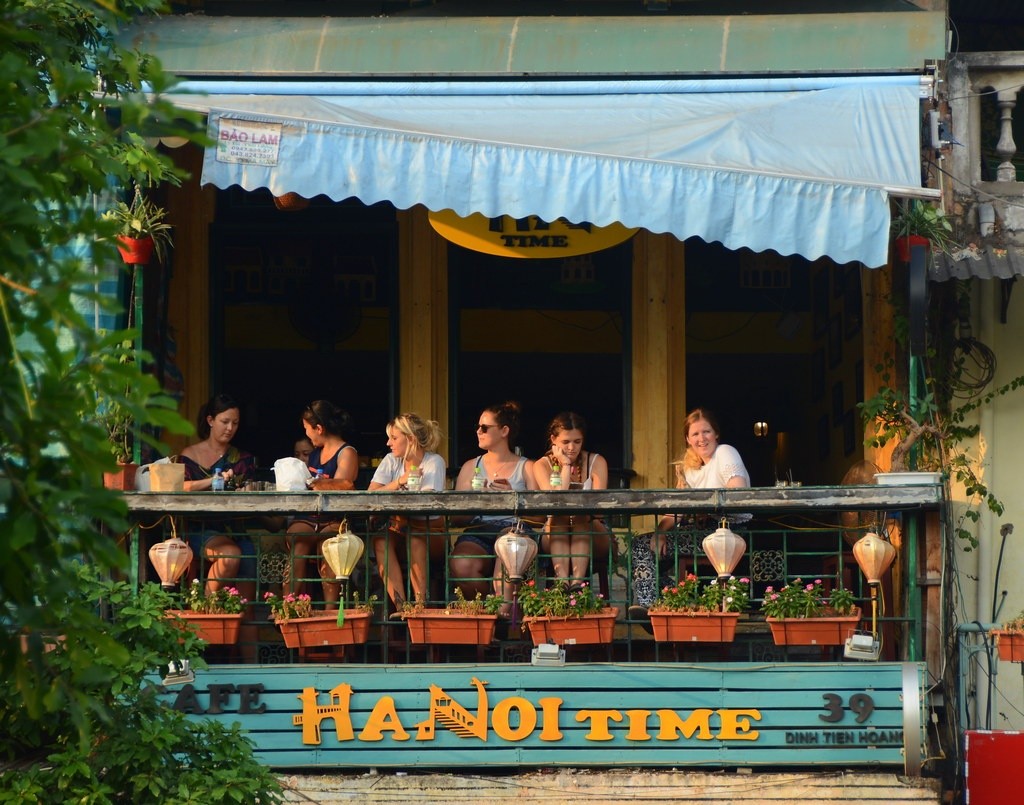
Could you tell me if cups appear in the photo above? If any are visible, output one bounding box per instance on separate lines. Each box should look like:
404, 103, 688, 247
775, 481, 801, 487
245, 481, 276, 491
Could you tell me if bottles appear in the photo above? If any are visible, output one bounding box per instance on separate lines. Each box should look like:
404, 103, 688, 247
471, 468, 484, 491
315, 469, 323, 479
407, 465, 419, 492
212, 468, 224, 492
549, 465, 562, 491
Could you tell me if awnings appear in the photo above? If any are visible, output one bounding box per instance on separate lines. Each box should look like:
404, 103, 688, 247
71, 71, 942, 269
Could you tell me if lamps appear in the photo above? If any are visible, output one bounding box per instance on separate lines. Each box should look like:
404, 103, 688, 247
531, 643, 565, 667
843, 634, 880, 660
162, 659, 195, 685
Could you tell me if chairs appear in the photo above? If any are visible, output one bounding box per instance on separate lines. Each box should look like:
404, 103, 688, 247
138, 469, 767, 637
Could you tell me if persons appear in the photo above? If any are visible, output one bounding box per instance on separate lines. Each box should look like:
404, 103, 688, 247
177, 394, 264, 664
628, 408, 754, 632
449, 401, 618, 620
269, 399, 445, 631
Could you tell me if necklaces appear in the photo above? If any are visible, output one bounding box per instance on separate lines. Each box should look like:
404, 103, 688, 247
485, 452, 512, 477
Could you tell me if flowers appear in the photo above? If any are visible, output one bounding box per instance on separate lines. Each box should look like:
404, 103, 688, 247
655, 573, 751, 613
760, 578, 862, 622
390, 588, 513, 615
517, 579, 604, 621
180, 578, 247, 613
264, 590, 378, 623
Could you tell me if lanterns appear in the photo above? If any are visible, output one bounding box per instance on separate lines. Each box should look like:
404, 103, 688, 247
149, 538, 193, 586
494, 519, 538, 631
853, 532, 895, 588
322, 519, 365, 628
702, 517, 746, 614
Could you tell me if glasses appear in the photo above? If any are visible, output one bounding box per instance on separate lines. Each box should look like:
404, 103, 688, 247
474, 424, 503, 433
304, 403, 323, 424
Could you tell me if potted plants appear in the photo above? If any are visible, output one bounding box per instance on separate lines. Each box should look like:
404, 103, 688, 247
855, 202, 974, 485
85, 173, 193, 493
988, 609, 1024, 661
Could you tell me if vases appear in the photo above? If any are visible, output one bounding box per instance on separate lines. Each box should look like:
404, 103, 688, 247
402, 609, 498, 644
766, 603, 861, 645
275, 606, 374, 649
647, 606, 741, 641
523, 606, 619, 644
152, 610, 244, 644
